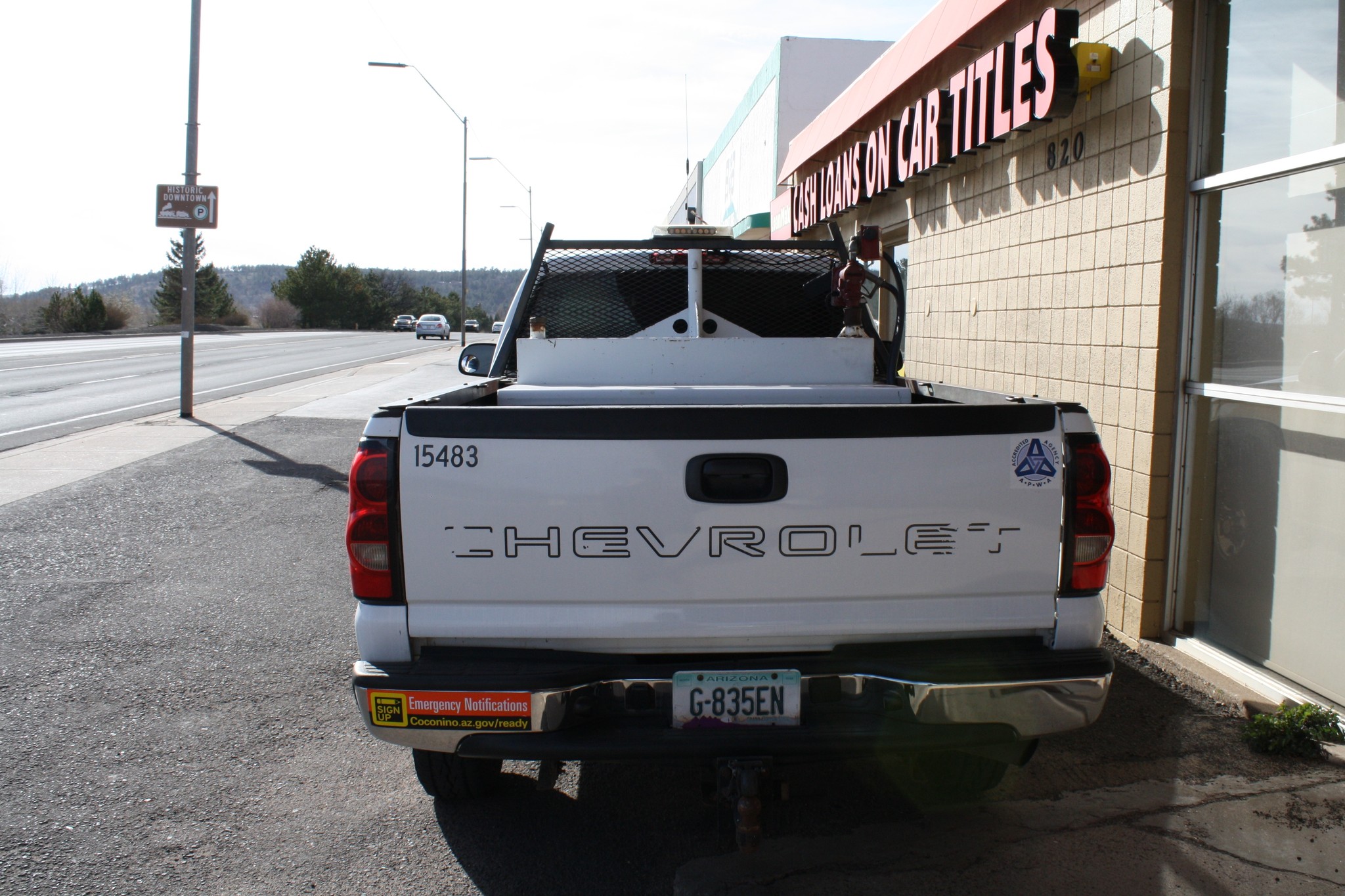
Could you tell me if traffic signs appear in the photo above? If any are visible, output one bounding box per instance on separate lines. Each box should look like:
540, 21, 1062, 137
155, 183, 219, 229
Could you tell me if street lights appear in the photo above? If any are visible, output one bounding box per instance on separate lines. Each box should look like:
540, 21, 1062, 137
469, 156, 532, 267
369, 61, 465, 348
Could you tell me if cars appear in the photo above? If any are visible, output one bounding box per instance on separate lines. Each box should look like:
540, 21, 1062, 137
392, 314, 417, 332
415, 313, 450, 340
492, 321, 504, 333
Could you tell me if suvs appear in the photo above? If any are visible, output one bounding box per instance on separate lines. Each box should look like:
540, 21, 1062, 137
464, 319, 480, 333
400, 320, 401, 324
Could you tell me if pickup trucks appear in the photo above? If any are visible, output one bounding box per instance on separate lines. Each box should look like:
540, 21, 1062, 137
345, 222, 1116, 809
